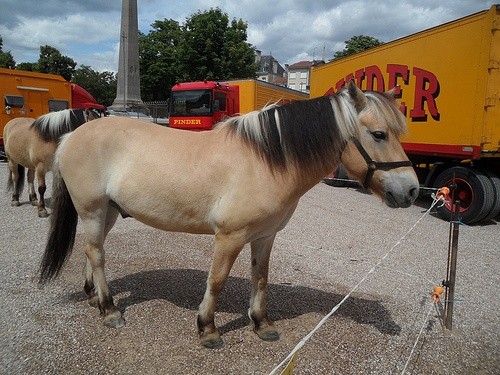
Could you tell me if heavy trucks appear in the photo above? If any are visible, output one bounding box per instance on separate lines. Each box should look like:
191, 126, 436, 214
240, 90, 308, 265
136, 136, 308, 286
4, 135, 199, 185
167, 80, 308, 132
0, 68, 108, 163
309, 3, 500, 225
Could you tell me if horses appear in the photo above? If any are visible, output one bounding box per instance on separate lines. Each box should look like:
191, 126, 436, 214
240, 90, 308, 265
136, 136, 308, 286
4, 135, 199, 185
30, 74, 421, 348
4, 106, 101, 219
